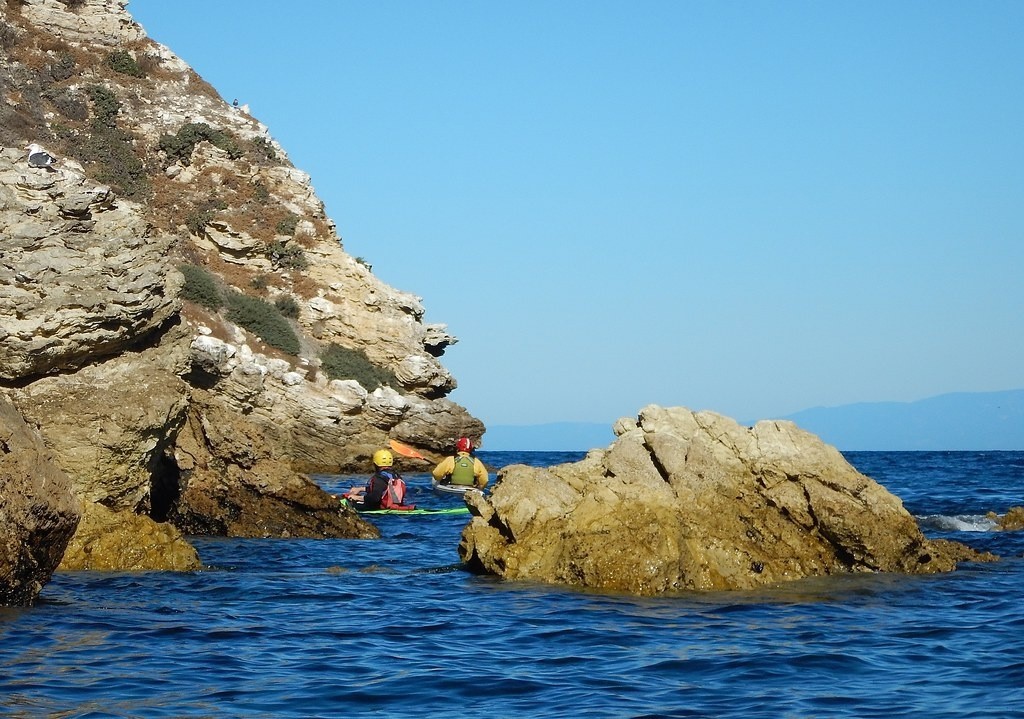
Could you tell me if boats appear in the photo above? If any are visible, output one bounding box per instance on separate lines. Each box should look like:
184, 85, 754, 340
341, 498, 469, 515
431, 477, 485, 498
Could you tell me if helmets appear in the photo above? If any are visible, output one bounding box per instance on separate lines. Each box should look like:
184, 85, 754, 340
455, 437, 472, 454
373, 450, 394, 467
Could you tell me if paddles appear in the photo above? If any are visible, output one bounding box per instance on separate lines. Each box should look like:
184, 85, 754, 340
345, 479, 359, 510
390, 440, 438, 466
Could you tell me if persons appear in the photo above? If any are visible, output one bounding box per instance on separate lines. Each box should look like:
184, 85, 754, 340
231, 98, 239, 109
432, 437, 489, 491
347, 449, 406, 512
